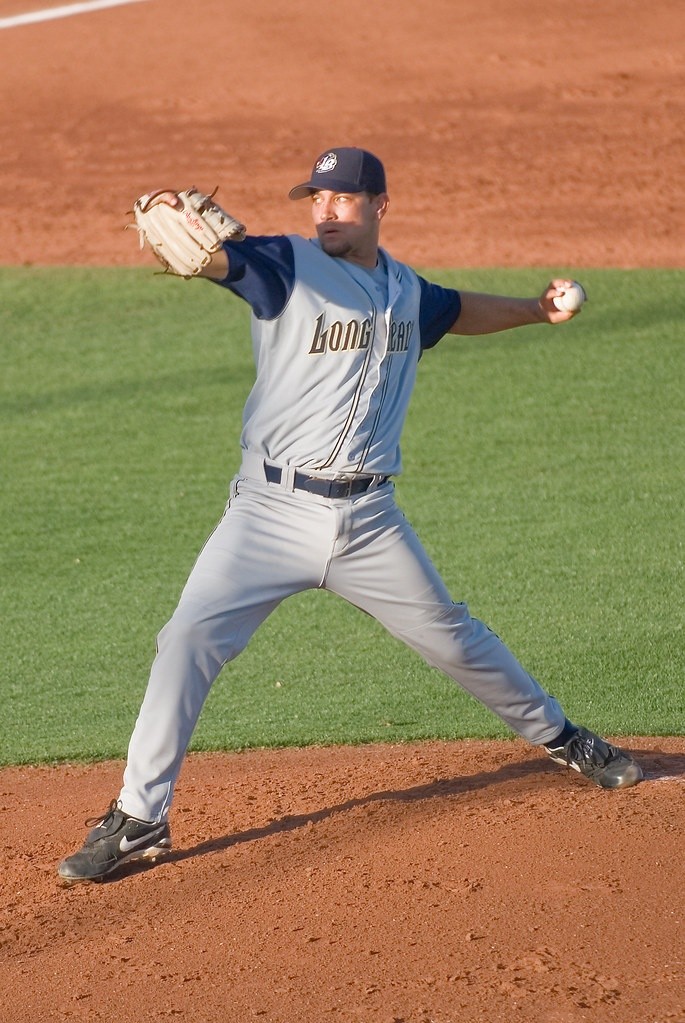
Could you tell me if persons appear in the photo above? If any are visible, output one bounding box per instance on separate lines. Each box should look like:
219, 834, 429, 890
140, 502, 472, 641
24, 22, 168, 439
59, 148, 645, 882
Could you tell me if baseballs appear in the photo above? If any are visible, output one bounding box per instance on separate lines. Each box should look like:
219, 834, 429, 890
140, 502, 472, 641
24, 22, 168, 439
553, 281, 585, 313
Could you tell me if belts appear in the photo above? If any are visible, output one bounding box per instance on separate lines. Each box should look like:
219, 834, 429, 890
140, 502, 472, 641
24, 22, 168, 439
265, 464, 389, 499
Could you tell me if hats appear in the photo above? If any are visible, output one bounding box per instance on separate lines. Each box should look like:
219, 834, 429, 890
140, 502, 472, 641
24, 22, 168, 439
288, 146, 388, 200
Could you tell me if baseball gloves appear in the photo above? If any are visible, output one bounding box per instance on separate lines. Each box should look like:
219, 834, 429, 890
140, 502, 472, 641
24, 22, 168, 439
134, 188, 246, 279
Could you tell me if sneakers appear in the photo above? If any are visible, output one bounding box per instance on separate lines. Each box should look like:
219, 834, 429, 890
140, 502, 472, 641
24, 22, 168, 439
57, 808, 173, 880
543, 719, 643, 789
